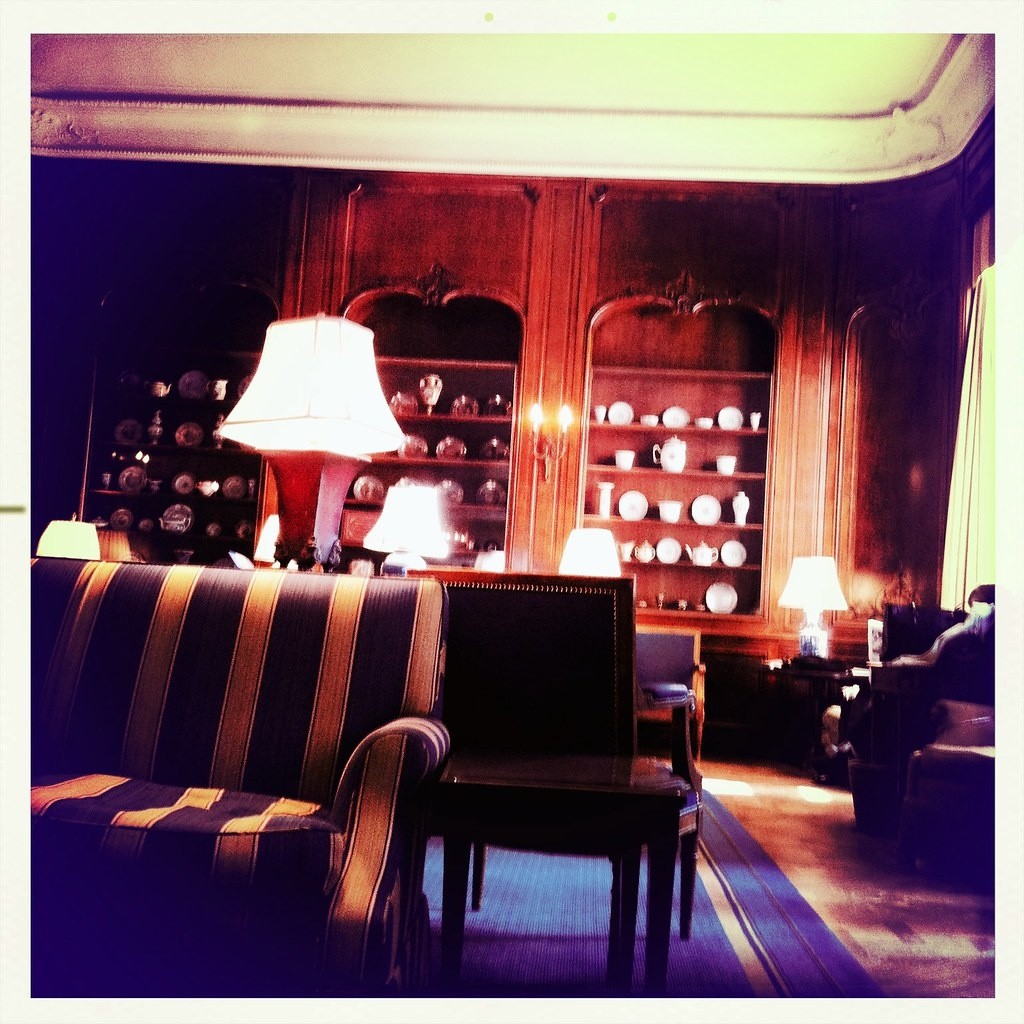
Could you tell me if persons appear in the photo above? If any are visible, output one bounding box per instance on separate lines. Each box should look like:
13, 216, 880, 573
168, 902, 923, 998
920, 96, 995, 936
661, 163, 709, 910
815, 584, 995, 783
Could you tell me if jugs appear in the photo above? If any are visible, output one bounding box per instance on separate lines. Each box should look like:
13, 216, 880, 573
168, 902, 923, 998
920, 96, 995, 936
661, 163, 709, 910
206, 380, 229, 400
653, 435, 686, 471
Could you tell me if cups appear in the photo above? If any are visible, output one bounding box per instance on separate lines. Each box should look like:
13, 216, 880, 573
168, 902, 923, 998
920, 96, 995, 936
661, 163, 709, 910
594, 405, 606, 424
750, 412, 761, 429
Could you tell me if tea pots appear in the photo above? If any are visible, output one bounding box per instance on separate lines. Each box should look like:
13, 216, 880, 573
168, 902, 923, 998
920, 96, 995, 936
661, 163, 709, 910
144, 382, 172, 396
685, 539, 718, 559
686, 544, 718, 566
635, 540, 655, 563
159, 518, 191, 534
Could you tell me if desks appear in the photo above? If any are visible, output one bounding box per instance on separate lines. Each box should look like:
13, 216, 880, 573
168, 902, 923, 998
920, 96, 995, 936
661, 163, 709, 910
762, 665, 868, 769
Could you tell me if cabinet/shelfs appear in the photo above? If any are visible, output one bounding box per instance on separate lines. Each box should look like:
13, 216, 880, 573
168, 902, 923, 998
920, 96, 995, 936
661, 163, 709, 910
580, 365, 775, 622
337, 354, 518, 551
73, 337, 266, 568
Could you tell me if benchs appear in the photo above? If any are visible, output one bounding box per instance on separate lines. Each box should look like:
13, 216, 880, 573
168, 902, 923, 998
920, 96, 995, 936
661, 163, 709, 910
30, 558, 705, 999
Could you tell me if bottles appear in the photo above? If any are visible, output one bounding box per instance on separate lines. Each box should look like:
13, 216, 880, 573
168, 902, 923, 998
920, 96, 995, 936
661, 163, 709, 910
732, 491, 750, 525
148, 411, 163, 445
212, 415, 226, 449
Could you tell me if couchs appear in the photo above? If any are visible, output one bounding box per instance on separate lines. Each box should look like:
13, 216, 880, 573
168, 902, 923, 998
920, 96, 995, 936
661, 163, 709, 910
847, 587, 994, 888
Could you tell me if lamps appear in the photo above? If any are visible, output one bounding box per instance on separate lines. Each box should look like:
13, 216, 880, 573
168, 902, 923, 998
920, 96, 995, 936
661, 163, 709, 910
215, 314, 406, 572
37, 520, 134, 563
559, 527, 622, 577
363, 481, 450, 578
780, 554, 849, 659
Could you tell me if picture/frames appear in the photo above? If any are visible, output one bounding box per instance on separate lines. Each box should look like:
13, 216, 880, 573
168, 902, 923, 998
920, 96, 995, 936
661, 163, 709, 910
868, 619, 883, 662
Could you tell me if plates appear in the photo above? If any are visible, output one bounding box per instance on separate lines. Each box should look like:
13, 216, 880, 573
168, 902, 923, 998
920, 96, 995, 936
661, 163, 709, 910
353, 393, 511, 551
91, 365, 255, 567
608, 401, 747, 614
